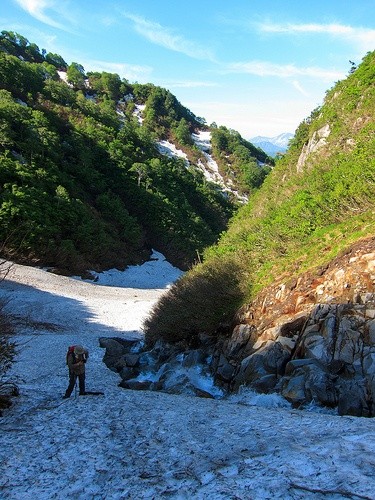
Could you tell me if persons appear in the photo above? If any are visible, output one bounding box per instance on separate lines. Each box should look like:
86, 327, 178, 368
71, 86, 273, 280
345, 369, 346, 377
62, 345, 89, 398
